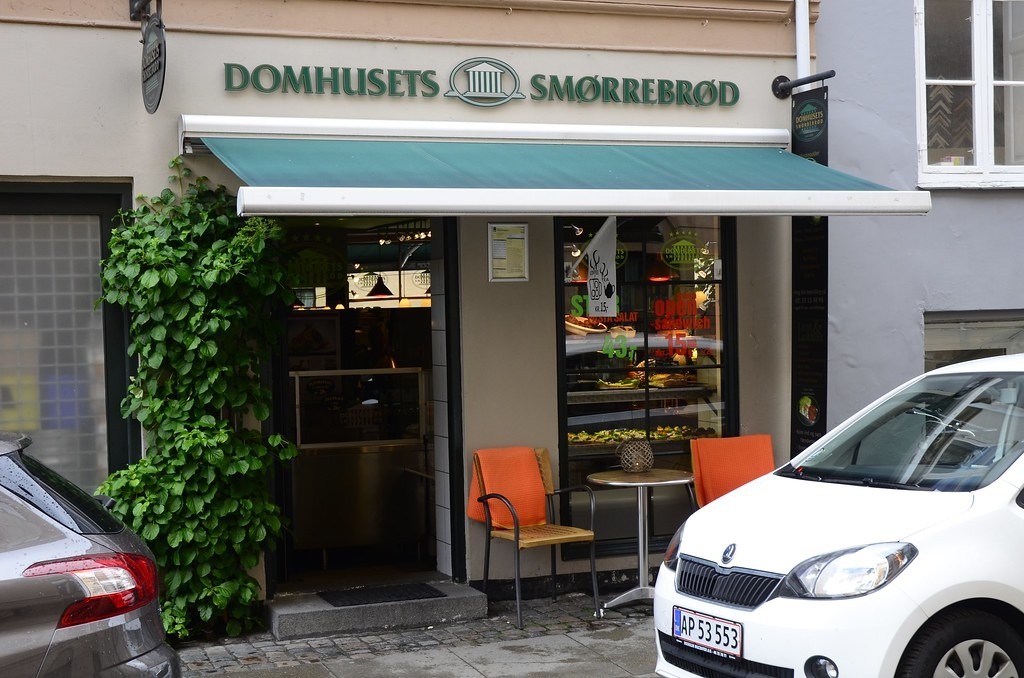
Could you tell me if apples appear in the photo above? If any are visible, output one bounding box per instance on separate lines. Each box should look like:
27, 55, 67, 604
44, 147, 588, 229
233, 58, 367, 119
566, 315, 605, 330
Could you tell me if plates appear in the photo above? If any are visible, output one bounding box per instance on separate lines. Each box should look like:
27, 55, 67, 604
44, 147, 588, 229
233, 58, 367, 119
564, 315, 607, 333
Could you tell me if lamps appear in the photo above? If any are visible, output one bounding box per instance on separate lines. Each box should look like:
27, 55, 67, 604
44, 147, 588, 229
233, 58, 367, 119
699, 299, 717, 311
564, 243, 581, 258
701, 240, 718, 255
376, 217, 432, 245
563, 223, 585, 236
566, 265, 582, 281
699, 260, 714, 278
317, 581, 446, 607
368, 241, 393, 296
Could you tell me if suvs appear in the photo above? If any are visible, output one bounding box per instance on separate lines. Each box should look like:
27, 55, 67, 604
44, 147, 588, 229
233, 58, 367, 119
0, 433, 183, 678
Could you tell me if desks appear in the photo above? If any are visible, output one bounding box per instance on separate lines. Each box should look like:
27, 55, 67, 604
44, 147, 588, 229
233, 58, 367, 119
586, 469, 695, 609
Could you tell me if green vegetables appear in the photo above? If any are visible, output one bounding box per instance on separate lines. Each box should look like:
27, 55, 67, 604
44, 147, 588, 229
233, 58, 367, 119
599, 379, 639, 386
568, 425, 691, 443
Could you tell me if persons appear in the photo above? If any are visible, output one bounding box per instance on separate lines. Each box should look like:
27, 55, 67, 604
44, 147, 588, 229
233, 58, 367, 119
366, 323, 397, 369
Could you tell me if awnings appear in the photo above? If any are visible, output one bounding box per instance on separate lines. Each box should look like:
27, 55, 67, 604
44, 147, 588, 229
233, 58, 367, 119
198, 136, 935, 217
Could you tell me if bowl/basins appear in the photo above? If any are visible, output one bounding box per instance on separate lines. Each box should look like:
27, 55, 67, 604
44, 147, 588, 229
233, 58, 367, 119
608, 329, 636, 339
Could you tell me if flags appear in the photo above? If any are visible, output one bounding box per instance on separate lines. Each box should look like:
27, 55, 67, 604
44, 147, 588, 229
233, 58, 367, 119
586, 220, 617, 317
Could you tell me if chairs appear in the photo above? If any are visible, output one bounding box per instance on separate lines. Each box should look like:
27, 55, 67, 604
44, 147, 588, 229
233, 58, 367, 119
686, 433, 774, 511
465, 446, 601, 631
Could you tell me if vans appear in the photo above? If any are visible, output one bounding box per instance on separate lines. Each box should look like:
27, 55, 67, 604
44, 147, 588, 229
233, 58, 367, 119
556, 335, 724, 433
650, 353, 1024, 677
